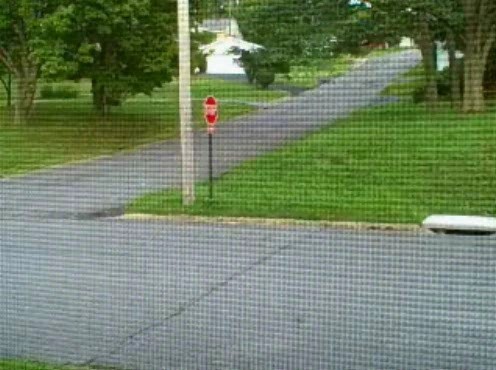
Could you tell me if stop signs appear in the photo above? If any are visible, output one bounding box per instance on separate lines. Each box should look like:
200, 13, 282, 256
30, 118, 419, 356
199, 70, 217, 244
204, 97, 218, 127
